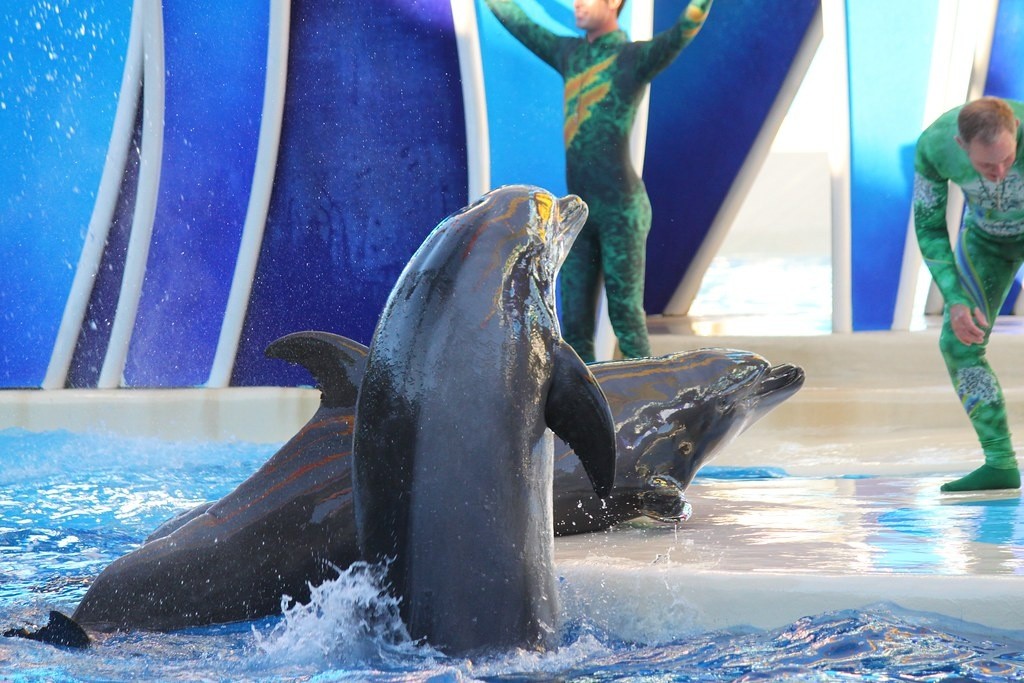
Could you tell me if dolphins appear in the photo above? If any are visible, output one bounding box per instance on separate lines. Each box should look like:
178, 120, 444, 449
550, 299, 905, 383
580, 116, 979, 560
2, 186, 807, 683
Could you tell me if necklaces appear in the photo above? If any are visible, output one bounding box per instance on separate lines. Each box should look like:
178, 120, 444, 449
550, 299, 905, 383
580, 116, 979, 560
979, 164, 1007, 209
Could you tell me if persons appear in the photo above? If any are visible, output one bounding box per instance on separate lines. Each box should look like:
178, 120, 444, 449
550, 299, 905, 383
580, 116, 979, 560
913, 94, 1024, 493
483, 0, 716, 363
